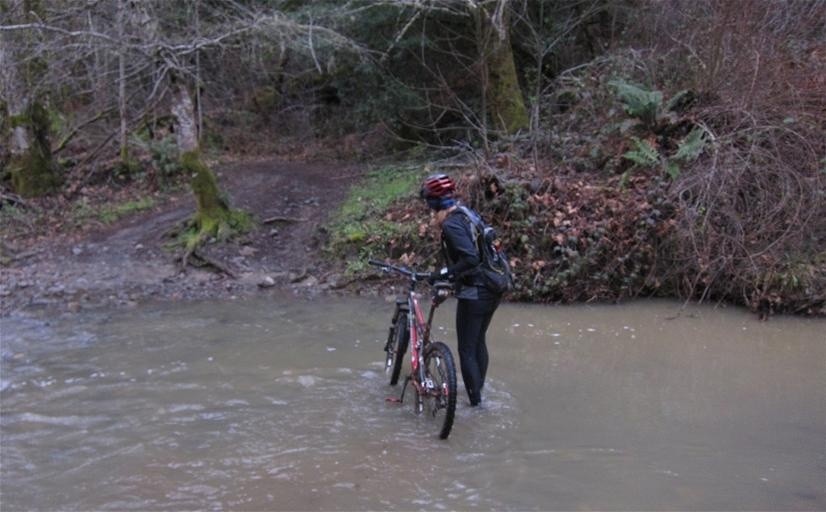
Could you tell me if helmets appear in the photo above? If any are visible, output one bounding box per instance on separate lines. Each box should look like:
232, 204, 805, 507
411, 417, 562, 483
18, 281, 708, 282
417, 174, 456, 204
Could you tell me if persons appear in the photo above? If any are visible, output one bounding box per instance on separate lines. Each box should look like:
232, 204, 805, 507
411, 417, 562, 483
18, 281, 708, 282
420, 172, 514, 407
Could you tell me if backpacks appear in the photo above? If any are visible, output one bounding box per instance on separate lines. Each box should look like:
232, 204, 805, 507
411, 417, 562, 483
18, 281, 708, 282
474, 222, 513, 295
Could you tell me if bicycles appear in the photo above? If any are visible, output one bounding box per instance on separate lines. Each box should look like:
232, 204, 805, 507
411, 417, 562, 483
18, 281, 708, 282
366, 258, 459, 437
